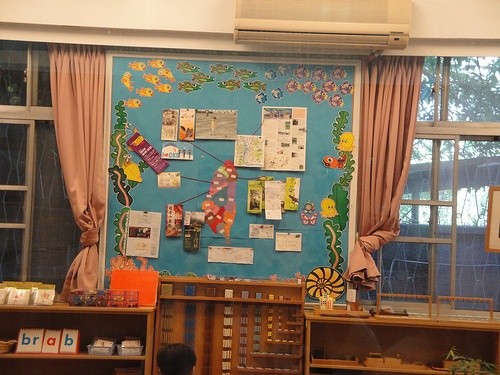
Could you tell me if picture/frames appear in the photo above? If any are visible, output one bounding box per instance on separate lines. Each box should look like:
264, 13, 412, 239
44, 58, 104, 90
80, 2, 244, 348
486, 186, 500, 253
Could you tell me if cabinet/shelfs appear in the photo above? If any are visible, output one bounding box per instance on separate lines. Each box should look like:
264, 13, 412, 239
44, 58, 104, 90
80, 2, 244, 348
304, 310, 500, 375
153, 276, 305, 375
0, 304, 157, 375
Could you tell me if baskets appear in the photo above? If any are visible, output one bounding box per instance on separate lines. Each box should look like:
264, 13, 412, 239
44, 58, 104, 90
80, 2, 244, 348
87, 343, 115, 357
117, 344, 143, 356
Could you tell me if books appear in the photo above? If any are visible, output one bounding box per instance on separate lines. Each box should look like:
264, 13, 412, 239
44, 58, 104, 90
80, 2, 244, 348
0, 281, 56, 306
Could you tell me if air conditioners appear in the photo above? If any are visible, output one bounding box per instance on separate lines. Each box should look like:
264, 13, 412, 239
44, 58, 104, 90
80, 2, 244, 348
233, 0, 412, 50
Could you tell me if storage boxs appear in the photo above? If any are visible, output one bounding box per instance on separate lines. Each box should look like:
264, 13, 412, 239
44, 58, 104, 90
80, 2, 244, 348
441, 360, 480, 370
310, 354, 358, 365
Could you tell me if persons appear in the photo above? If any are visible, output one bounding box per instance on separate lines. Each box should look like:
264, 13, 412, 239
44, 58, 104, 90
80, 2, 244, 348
157, 343, 196, 375
